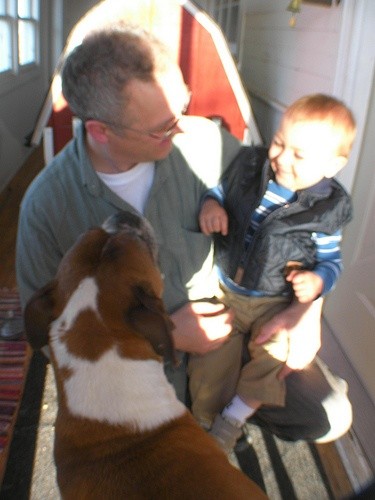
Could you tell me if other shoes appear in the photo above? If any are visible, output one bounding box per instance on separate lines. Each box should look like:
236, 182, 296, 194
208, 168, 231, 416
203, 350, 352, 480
188, 414, 216, 434
203, 414, 241, 458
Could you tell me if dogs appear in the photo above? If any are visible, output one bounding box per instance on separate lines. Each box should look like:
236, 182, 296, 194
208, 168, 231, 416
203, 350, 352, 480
23, 209, 269, 500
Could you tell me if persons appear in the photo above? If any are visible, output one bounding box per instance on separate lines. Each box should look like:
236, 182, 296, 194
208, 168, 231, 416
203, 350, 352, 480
187, 94, 356, 455
15, 22, 356, 455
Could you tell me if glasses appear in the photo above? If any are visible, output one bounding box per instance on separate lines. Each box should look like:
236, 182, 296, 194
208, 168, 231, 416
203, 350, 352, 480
90, 88, 194, 143
